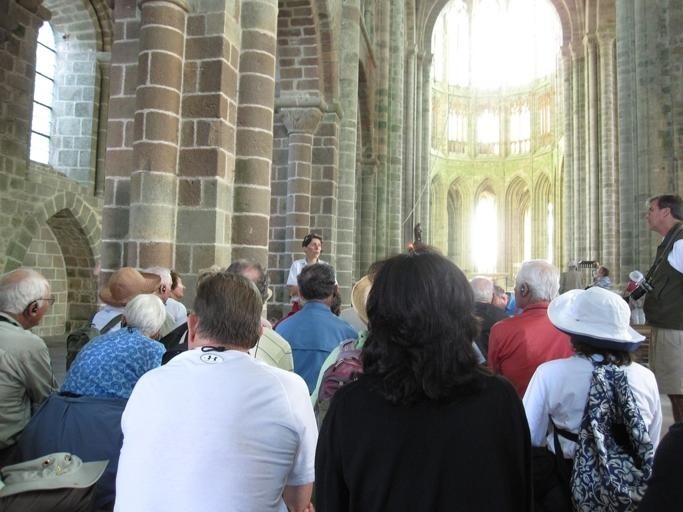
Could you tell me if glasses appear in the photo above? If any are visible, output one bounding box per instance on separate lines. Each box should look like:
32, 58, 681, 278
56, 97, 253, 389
27, 294, 55, 309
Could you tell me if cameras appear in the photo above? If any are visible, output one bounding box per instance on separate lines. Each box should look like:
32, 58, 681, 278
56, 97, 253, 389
630, 277, 655, 300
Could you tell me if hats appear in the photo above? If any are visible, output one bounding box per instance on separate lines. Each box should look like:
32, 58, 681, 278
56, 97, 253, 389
98, 267, 161, 306
547, 286, 646, 344
350, 272, 375, 324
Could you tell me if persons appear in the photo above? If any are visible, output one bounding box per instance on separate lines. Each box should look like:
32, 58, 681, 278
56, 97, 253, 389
644, 194, 682, 425
286, 234, 339, 311
0, 244, 682, 511
